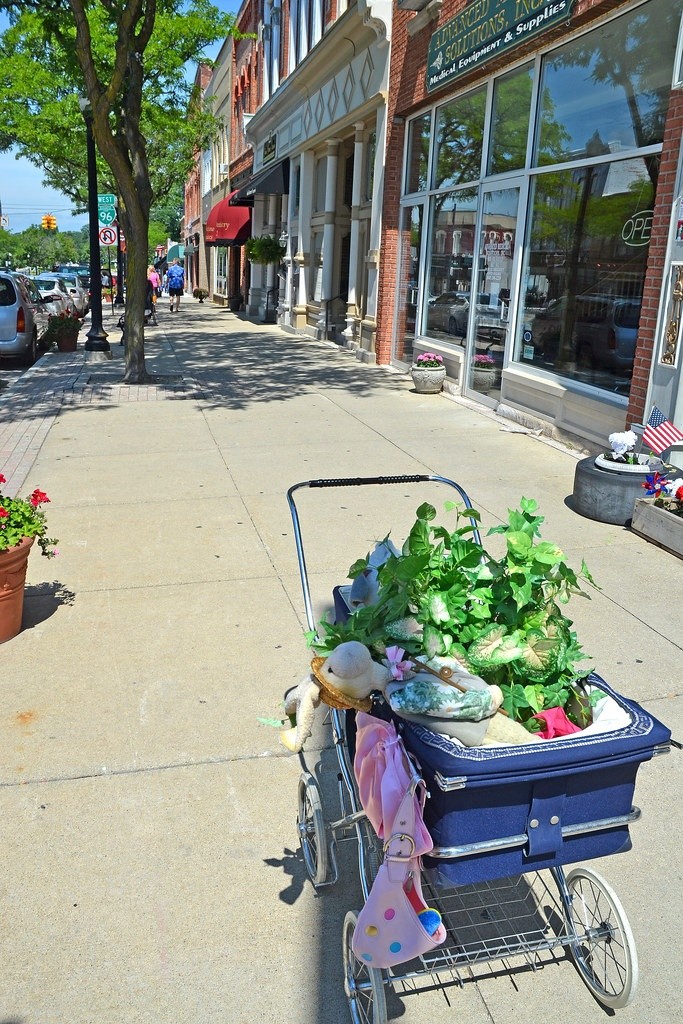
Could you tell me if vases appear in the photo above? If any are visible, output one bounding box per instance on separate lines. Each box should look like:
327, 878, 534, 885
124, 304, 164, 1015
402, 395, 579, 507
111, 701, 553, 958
630, 496, 683, 560
471, 367, 495, 393
57, 333, 77, 352
0, 536, 34, 645
411, 364, 447, 394
594, 452, 665, 476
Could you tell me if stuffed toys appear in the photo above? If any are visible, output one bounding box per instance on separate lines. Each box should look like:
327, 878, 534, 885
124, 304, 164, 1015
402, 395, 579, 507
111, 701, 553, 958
278, 637, 390, 757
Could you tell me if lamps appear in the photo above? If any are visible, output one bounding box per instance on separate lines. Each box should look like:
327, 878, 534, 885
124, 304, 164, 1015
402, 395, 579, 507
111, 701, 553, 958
278, 230, 288, 247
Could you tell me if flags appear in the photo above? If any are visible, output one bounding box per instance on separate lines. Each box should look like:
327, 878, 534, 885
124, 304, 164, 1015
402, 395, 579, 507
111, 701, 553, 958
643, 404, 683, 457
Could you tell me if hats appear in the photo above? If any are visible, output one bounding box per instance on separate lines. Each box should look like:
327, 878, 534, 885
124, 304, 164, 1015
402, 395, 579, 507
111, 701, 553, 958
321, 641, 389, 696
311, 655, 375, 712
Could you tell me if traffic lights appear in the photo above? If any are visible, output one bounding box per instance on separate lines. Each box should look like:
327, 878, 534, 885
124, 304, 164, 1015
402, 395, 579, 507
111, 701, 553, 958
47, 216, 53, 229
41, 216, 48, 231
50, 217, 57, 229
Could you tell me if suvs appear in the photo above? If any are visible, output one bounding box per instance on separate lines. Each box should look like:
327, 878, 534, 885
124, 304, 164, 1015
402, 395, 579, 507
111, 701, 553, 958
0, 267, 54, 365
532, 293, 641, 375
53, 265, 91, 289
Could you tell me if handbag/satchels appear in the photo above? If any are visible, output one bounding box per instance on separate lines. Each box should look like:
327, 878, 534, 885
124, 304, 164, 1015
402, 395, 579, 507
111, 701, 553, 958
352, 776, 447, 970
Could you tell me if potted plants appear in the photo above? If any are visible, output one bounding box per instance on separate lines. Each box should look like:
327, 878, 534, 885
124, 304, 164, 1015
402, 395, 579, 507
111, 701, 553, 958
193, 288, 209, 303
105, 288, 117, 302
228, 294, 244, 311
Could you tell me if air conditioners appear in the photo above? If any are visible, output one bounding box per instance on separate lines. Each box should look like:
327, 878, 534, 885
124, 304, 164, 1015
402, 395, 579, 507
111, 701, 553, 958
219, 164, 228, 175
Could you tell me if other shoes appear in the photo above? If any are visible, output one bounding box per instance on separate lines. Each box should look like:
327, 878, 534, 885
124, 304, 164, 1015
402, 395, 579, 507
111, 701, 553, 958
176, 308, 179, 311
169, 304, 174, 312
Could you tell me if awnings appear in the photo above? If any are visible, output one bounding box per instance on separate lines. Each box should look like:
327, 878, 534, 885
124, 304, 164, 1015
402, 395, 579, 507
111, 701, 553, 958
228, 156, 290, 208
165, 244, 185, 263
203, 188, 252, 247
183, 242, 199, 255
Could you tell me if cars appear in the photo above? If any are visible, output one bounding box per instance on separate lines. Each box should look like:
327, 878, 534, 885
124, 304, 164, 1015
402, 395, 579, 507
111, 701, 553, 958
423, 290, 509, 336
28, 276, 78, 319
38, 271, 90, 317
408, 286, 439, 304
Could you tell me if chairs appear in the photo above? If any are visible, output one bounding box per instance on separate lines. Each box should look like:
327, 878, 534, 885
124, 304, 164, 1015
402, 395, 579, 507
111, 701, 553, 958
64, 282, 74, 287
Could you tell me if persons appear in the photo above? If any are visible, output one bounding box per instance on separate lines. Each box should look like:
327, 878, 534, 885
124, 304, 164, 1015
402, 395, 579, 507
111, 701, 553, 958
163, 257, 185, 312
101, 271, 110, 289
148, 264, 161, 313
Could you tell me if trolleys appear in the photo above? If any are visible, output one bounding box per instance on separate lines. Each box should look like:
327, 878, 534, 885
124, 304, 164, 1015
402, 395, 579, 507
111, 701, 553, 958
282, 474, 681, 1024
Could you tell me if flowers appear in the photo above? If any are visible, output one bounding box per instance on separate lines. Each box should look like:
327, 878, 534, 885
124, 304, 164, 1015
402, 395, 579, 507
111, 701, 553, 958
0, 473, 61, 560
42, 306, 86, 351
641, 470, 683, 518
472, 354, 496, 368
416, 353, 443, 368
605, 429, 653, 465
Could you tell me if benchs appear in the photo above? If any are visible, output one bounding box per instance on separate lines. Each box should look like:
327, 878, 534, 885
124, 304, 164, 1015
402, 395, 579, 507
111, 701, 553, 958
117, 307, 152, 346
460, 331, 501, 355
